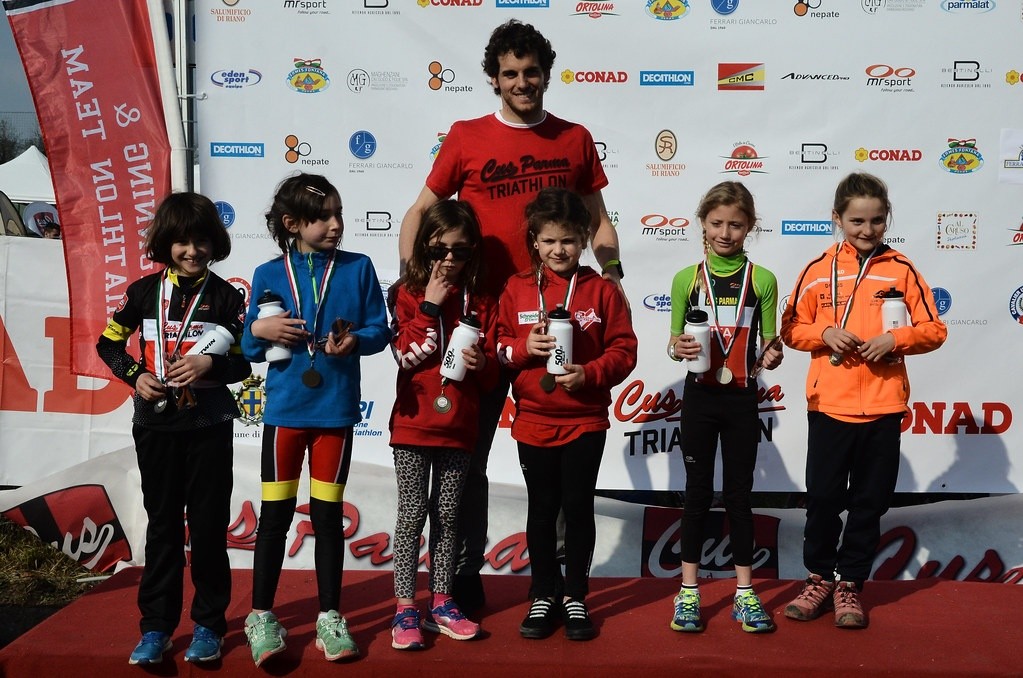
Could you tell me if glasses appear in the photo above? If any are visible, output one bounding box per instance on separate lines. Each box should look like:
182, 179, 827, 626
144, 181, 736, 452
426, 244, 475, 262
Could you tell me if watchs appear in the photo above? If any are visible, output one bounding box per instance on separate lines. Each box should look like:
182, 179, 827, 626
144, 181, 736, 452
668, 342, 682, 362
602, 260, 624, 278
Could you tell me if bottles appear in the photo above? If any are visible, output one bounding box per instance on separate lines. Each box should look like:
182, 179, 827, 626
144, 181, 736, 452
545, 304, 573, 374
881, 286, 907, 333
439, 314, 482, 381
684, 307, 711, 373
184, 322, 238, 355
256, 289, 292, 362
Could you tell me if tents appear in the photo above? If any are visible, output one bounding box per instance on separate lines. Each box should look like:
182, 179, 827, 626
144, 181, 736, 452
0, 145, 57, 203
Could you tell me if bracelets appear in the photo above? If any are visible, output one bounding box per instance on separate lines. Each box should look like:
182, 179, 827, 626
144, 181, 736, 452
419, 300, 442, 317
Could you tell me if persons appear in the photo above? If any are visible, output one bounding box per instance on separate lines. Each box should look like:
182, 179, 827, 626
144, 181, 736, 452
780, 173, 948, 628
95, 191, 252, 667
399, 18, 620, 634
43, 222, 60, 239
496, 185, 637, 639
241, 173, 393, 668
668, 180, 784, 634
385, 200, 502, 640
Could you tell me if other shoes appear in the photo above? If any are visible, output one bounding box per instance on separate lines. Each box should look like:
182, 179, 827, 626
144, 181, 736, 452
453, 591, 486, 620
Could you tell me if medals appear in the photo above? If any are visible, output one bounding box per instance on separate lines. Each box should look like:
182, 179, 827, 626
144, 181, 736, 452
540, 373, 556, 393
302, 367, 322, 387
154, 397, 167, 413
829, 350, 843, 366
716, 367, 732, 385
433, 393, 451, 414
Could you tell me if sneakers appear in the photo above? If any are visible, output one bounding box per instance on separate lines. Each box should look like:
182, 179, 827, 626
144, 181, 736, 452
833, 581, 868, 628
519, 595, 561, 638
731, 591, 774, 632
129, 630, 172, 665
423, 597, 479, 639
184, 623, 224, 662
391, 608, 425, 648
784, 573, 836, 621
559, 597, 596, 641
244, 611, 287, 669
669, 590, 701, 631
315, 609, 358, 661
